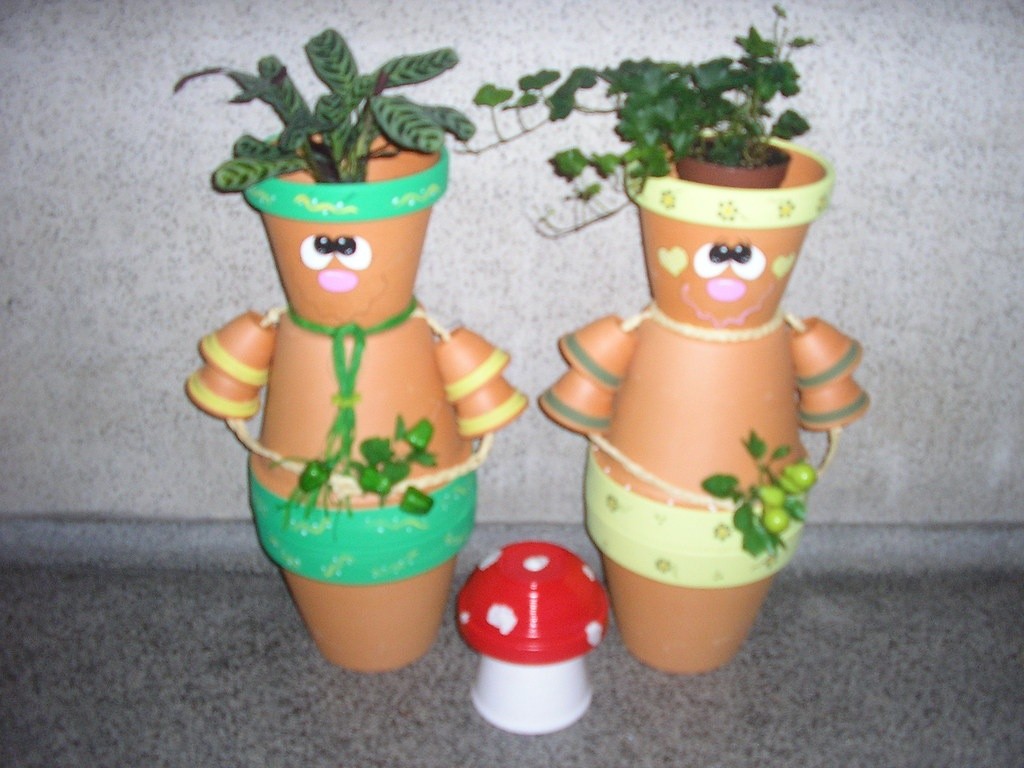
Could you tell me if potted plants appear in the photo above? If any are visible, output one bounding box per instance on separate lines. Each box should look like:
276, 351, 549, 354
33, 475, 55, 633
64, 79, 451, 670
467, 6, 811, 237
